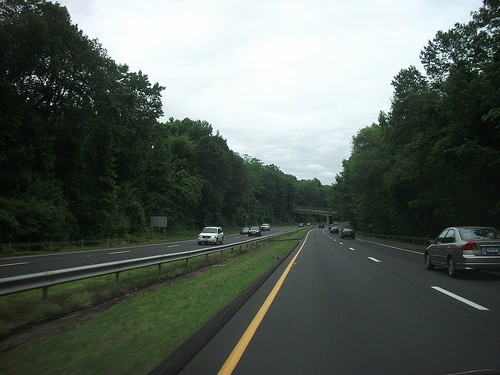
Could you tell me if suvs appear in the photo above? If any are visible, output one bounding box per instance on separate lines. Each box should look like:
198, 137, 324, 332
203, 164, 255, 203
248, 226, 261, 237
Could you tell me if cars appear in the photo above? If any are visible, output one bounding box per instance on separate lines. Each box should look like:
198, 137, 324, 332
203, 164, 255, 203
260, 224, 270, 231
424, 227, 500, 278
340, 227, 355, 239
330, 227, 339, 234
197, 227, 224, 245
240, 227, 250, 234
